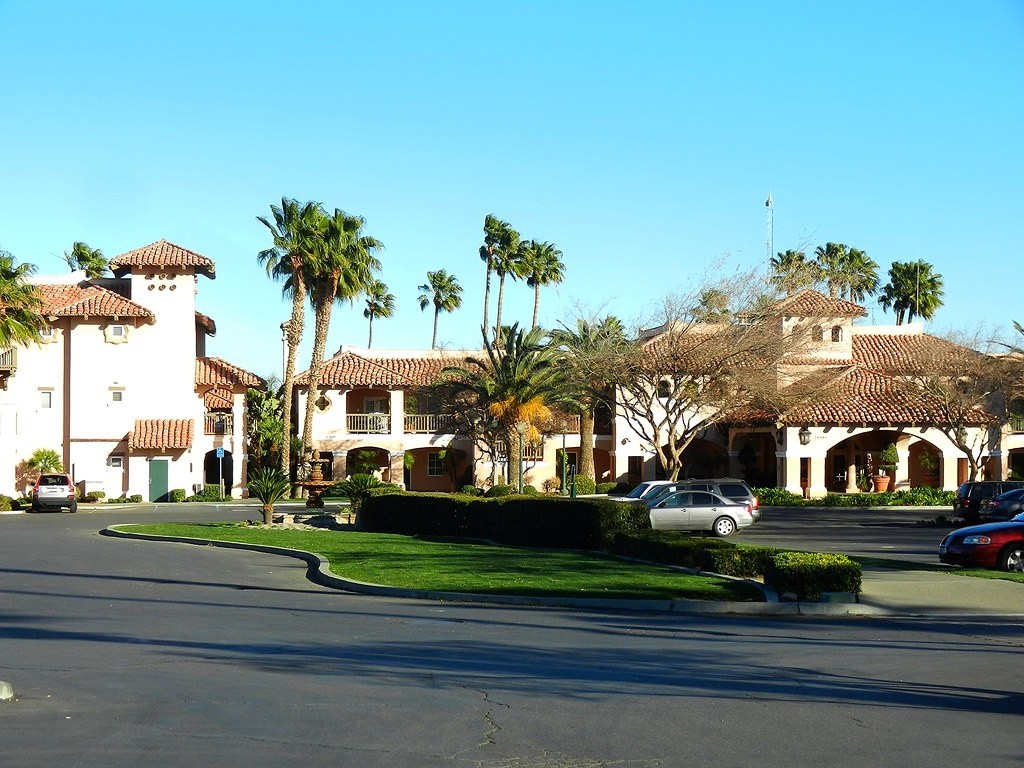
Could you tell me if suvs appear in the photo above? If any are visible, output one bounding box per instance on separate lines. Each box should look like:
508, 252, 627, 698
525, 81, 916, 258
31, 473, 78, 513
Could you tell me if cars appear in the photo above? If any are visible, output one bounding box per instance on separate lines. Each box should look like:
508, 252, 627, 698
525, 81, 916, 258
938, 511, 1024, 574
952, 480, 1024, 524
632, 489, 756, 538
628, 479, 764, 524
610, 481, 677, 501
978, 487, 1024, 521
